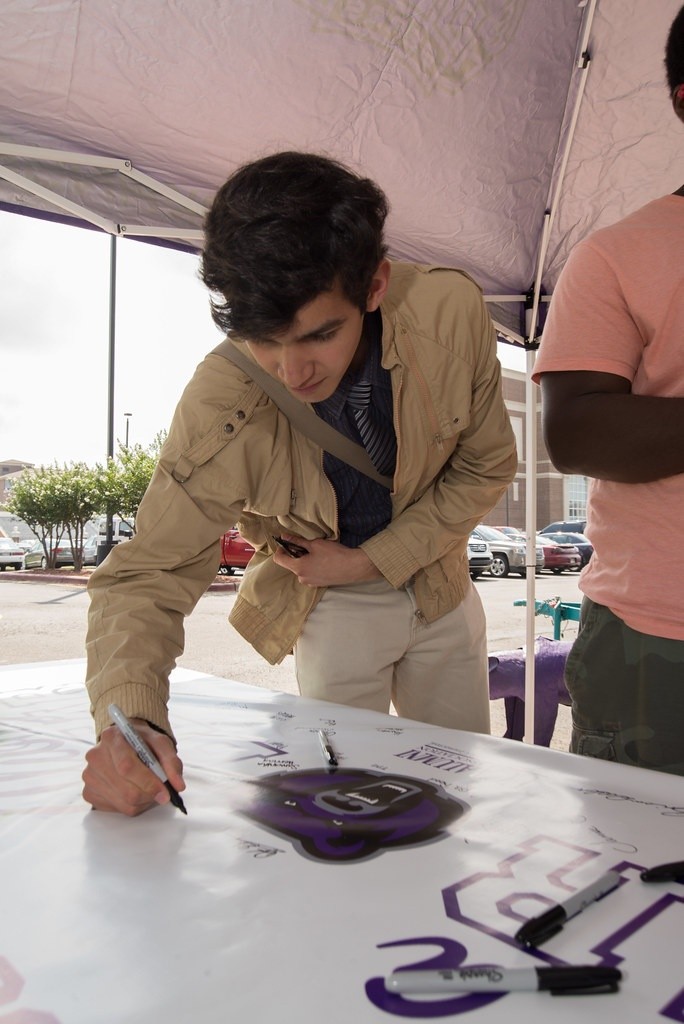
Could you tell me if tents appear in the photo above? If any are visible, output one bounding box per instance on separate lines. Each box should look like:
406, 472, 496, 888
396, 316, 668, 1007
1, 0, 684, 744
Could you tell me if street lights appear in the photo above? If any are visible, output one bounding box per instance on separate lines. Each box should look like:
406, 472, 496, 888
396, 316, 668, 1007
123, 412, 133, 448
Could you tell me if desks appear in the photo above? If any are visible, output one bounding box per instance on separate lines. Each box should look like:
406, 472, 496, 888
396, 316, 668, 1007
0, 654, 684, 1024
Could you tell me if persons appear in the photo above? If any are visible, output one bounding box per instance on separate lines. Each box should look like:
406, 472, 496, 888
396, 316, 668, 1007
529, 4, 684, 777
82, 152, 517, 817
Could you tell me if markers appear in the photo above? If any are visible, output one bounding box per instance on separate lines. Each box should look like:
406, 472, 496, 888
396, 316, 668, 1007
385, 965, 622, 997
640, 859, 684, 883
318, 729, 337, 764
109, 704, 189, 816
515, 872, 620, 947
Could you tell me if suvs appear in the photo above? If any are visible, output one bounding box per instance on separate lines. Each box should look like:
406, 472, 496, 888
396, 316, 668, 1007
467, 538, 494, 579
472, 525, 546, 578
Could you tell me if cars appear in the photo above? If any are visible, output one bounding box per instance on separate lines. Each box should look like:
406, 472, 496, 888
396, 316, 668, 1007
0, 515, 256, 575
493, 520, 590, 575
